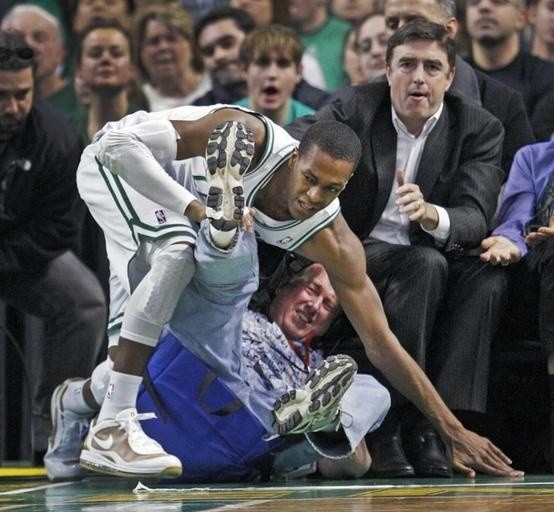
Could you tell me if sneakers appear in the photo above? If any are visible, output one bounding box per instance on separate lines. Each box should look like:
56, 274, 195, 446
43, 377, 93, 482
80, 408, 183, 478
268, 354, 358, 436
204, 121, 255, 232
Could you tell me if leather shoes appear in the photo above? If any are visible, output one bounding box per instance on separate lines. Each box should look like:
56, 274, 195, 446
370, 425, 415, 477
418, 431, 451, 477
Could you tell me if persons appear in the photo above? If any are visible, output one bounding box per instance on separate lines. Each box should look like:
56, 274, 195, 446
1, 0, 553, 476
43, 95, 524, 474
135, 120, 393, 473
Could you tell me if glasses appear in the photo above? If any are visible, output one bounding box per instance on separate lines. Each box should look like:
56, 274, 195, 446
1, 47, 35, 61
